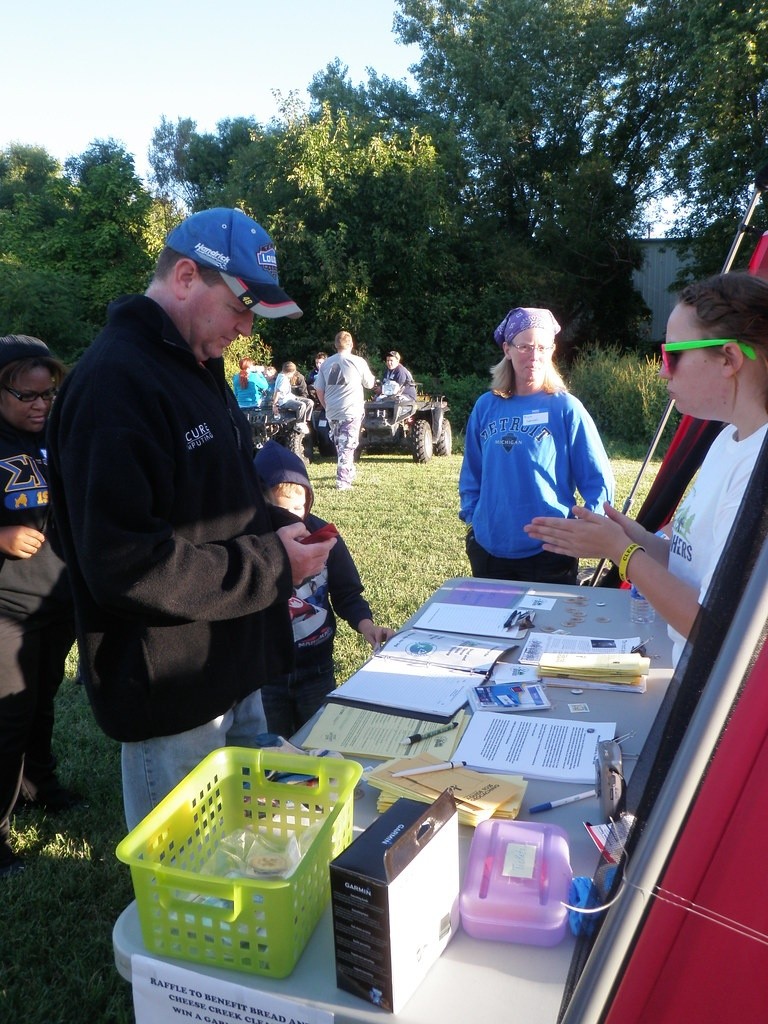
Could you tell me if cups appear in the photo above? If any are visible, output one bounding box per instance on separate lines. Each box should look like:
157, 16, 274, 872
274, 412, 281, 420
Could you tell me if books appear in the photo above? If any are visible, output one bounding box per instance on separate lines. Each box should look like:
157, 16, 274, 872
299, 601, 650, 828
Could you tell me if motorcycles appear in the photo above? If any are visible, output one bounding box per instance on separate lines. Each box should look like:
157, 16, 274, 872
362, 382, 453, 464
241, 406, 337, 465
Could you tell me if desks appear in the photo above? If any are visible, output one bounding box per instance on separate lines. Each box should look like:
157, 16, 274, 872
114, 574, 673, 1024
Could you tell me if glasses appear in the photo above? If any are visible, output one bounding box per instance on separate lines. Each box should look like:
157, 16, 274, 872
511, 341, 554, 354
0, 382, 58, 403
661, 339, 757, 374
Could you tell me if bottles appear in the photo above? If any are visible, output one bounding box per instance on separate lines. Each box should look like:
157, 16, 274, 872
252, 366, 265, 372
629, 584, 655, 623
374, 379, 382, 386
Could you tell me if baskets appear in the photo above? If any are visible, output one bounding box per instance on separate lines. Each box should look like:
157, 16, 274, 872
114, 746, 363, 978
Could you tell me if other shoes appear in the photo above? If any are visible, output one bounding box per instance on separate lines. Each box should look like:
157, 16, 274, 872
293, 423, 310, 435
337, 481, 354, 491
32, 788, 85, 812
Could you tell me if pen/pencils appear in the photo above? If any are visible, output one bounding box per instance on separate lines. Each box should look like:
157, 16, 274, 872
405, 722, 459, 745
530, 789, 596, 814
504, 610, 521, 631
392, 761, 466, 777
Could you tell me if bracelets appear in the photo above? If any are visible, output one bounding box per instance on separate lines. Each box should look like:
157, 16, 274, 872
464, 522, 472, 533
619, 542, 645, 585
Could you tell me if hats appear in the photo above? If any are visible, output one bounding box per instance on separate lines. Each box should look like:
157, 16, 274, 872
165, 208, 303, 319
0, 333, 52, 370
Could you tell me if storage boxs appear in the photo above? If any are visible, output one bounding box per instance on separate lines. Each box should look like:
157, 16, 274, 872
459, 819, 576, 946
329, 789, 459, 1011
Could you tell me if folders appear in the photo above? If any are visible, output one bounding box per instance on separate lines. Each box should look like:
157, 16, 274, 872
325, 627, 519, 725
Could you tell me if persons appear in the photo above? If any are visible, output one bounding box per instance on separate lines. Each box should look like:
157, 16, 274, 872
272, 361, 314, 433
456, 307, 613, 579
47, 208, 337, 837
0, 333, 78, 867
527, 272, 768, 669
231, 358, 277, 423
309, 351, 328, 384
316, 330, 375, 491
252, 438, 396, 736
373, 351, 416, 417
